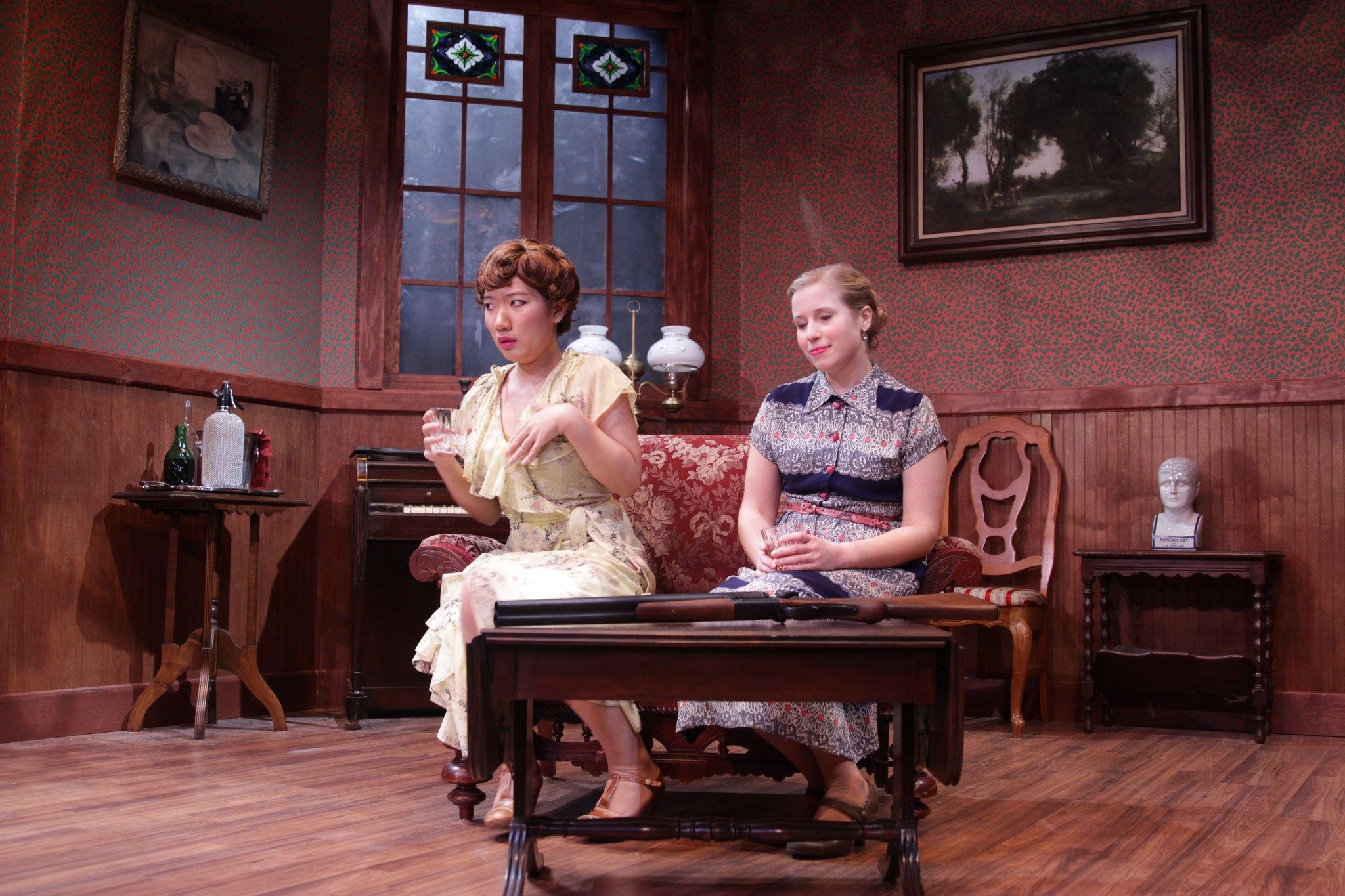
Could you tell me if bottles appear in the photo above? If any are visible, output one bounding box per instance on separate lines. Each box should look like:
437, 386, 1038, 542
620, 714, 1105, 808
164, 425, 195, 486
201, 381, 245, 488
182, 400, 198, 486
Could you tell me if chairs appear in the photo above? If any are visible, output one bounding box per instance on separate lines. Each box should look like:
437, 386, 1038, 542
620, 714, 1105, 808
938, 414, 1063, 740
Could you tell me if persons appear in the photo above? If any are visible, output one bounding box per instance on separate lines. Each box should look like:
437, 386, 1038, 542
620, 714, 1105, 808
1148, 455, 1203, 550
674, 262, 948, 858
411, 236, 666, 826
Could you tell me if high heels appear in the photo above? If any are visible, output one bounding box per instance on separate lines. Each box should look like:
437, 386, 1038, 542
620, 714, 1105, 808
578, 763, 664, 818
484, 762, 543, 828
786, 779, 880, 857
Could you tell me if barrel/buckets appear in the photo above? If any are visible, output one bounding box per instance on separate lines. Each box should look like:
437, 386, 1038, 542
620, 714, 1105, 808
196, 430, 261, 489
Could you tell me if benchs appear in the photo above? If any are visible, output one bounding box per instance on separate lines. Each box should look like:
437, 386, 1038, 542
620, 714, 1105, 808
408, 434, 986, 822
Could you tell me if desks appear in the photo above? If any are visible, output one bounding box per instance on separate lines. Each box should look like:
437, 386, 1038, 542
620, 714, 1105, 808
109, 490, 313, 740
1074, 548, 1287, 744
462, 620, 966, 896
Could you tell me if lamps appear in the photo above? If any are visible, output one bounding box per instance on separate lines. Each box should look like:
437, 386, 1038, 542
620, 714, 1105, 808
564, 300, 705, 429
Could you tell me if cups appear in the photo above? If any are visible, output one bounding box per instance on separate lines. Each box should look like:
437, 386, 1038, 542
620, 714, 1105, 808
761, 522, 804, 571
429, 407, 470, 454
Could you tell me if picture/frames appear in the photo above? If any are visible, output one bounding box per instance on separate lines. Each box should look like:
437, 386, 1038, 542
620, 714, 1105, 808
112, 0, 281, 215
897, 4, 1214, 266
